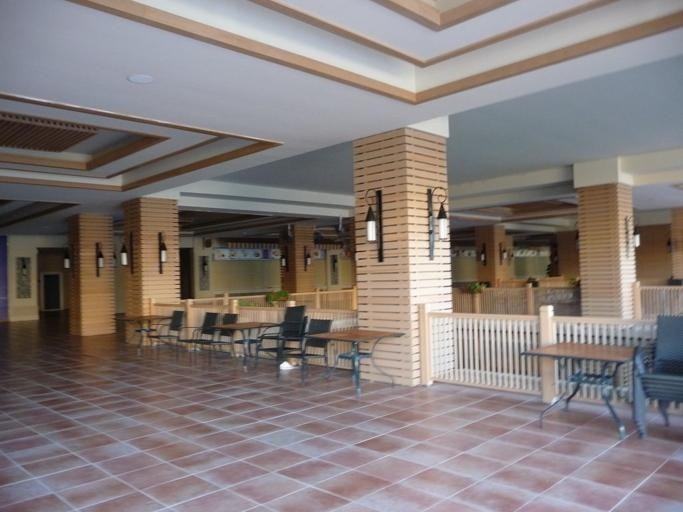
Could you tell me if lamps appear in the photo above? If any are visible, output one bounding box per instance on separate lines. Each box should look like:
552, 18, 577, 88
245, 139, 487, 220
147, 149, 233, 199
426, 186, 447, 260
119, 232, 133, 273
364, 188, 383, 263
158, 232, 167, 274
624, 215, 639, 256
280, 245, 310, 273
62, 241, 75, 278
201, 255, 207, 276
94, 241, 104, 278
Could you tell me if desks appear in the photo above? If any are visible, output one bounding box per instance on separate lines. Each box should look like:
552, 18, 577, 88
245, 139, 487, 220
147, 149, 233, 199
112, 314, 172, 349
521, 342, 635, 442
303, 329, 404, 398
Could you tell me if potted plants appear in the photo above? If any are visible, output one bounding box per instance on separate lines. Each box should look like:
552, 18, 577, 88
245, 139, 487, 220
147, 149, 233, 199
458, 281, 487, 294
265, 290, 288, 302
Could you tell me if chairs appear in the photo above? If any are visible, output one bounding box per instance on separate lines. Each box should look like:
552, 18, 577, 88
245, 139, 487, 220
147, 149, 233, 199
631, 314, 682, 438
133, 305, 334, 386
